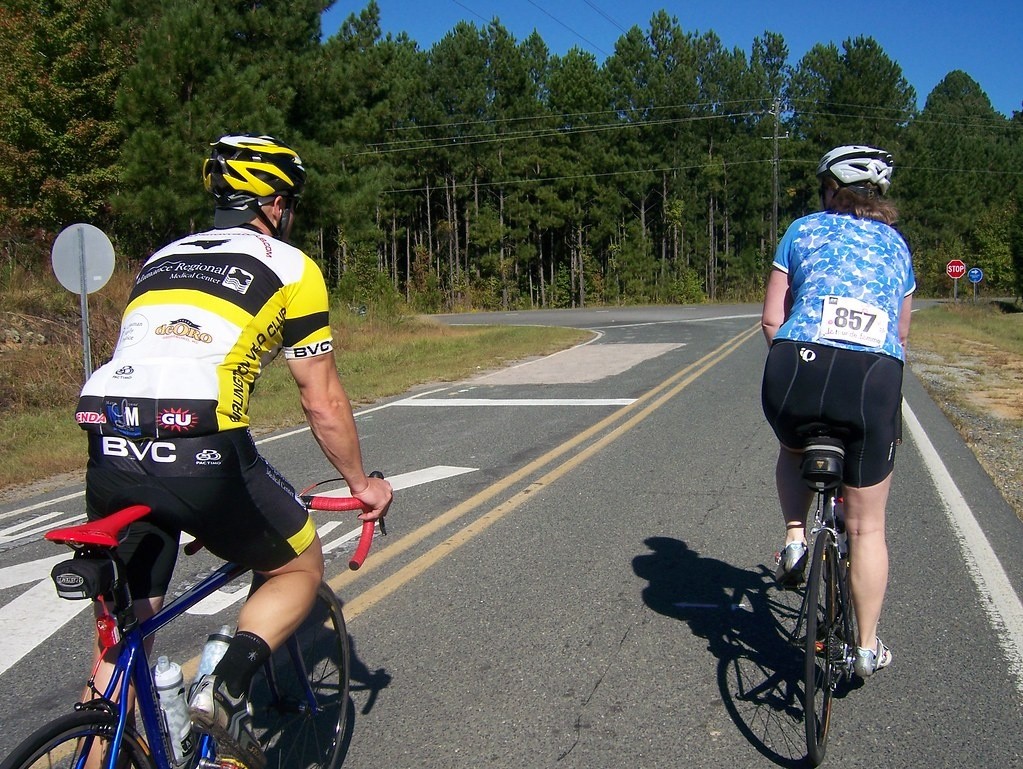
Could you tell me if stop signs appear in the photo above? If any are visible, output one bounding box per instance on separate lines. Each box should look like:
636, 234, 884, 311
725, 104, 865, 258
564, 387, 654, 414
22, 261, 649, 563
945, 259, 967, 279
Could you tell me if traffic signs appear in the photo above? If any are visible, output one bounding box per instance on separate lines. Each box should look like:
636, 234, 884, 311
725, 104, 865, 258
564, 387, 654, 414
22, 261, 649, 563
966, 267, 984, 283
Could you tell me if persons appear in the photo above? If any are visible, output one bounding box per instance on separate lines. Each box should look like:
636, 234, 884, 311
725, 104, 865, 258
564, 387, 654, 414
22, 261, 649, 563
761, 145, 916, 677
75, 135, 393, 769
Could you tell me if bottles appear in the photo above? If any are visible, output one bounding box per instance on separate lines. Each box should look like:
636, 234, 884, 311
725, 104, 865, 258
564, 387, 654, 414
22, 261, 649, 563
151, 656, 195, 763
192, 625, 233, 697
822, 518, 849, 583
836, 496, 847, 521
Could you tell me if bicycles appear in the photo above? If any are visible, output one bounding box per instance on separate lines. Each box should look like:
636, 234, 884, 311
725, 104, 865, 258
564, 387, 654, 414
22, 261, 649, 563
0, 469, 387, 769
777, 398, 911, 765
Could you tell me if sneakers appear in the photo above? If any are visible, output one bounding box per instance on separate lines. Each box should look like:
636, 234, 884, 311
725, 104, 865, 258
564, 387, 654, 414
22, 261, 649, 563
854, 636, 892, 676
775, 543, 809, 584
187, 673, 266, 769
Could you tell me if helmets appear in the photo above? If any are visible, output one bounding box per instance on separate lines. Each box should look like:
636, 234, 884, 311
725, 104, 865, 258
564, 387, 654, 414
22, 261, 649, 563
815, 145, 894, 195
202, 135, 308, 207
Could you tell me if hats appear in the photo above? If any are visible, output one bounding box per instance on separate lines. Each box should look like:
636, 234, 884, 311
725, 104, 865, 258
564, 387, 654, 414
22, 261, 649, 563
213, 195, 274, 228
